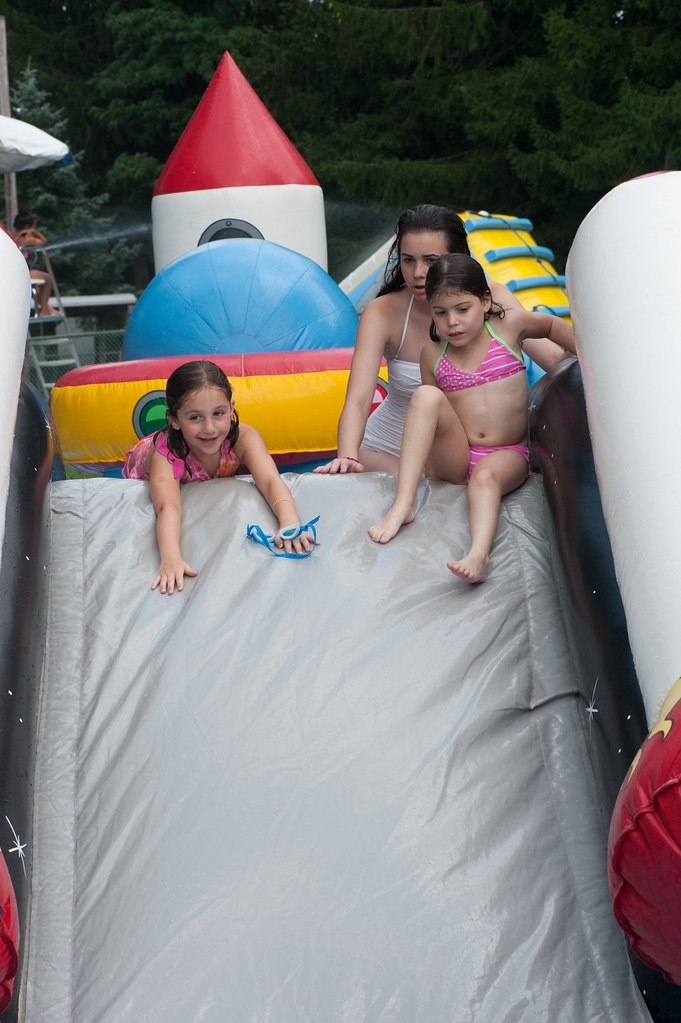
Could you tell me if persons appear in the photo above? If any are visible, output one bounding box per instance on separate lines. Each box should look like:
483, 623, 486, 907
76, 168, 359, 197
122, 359, 320, 595
10, 212, 59, 317
313, 205, 565, 476
368, 253, 577, 583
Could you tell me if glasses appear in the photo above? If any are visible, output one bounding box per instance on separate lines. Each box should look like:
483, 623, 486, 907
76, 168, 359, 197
248, 523, 303, 545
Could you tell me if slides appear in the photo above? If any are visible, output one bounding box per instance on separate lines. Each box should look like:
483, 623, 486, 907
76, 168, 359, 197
9, 467, 659, 1023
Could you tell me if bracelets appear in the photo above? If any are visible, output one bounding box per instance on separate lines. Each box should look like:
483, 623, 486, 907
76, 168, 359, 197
272, 499, 295, 514
343, 456, 359, 463
